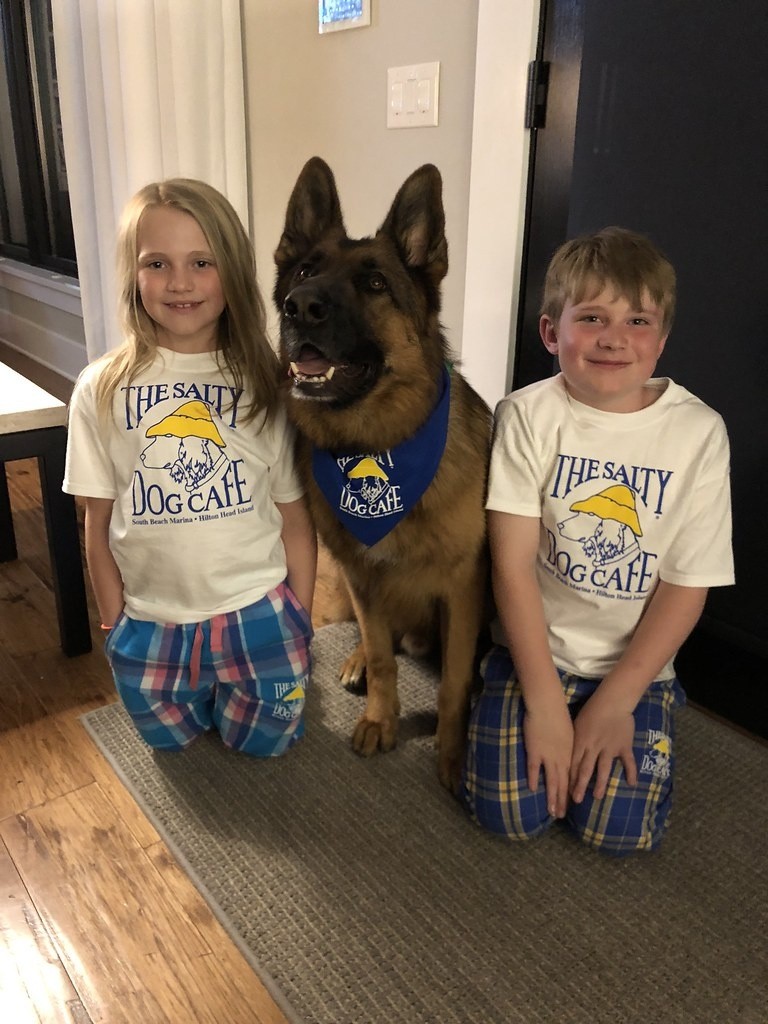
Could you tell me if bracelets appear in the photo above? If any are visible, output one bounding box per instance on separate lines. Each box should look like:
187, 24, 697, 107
100, 624, 113, 632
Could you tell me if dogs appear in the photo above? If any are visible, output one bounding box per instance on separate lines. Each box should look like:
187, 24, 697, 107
272, 157, 493, 798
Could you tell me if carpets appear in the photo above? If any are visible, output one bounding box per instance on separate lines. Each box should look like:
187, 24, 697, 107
82, 616, 768, 1024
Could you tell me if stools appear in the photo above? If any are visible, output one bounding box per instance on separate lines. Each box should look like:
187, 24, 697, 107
0, 362, 92, 655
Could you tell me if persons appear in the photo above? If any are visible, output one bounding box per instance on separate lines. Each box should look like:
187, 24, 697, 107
61, 177, 318, 757
461, 226, 735, 857
